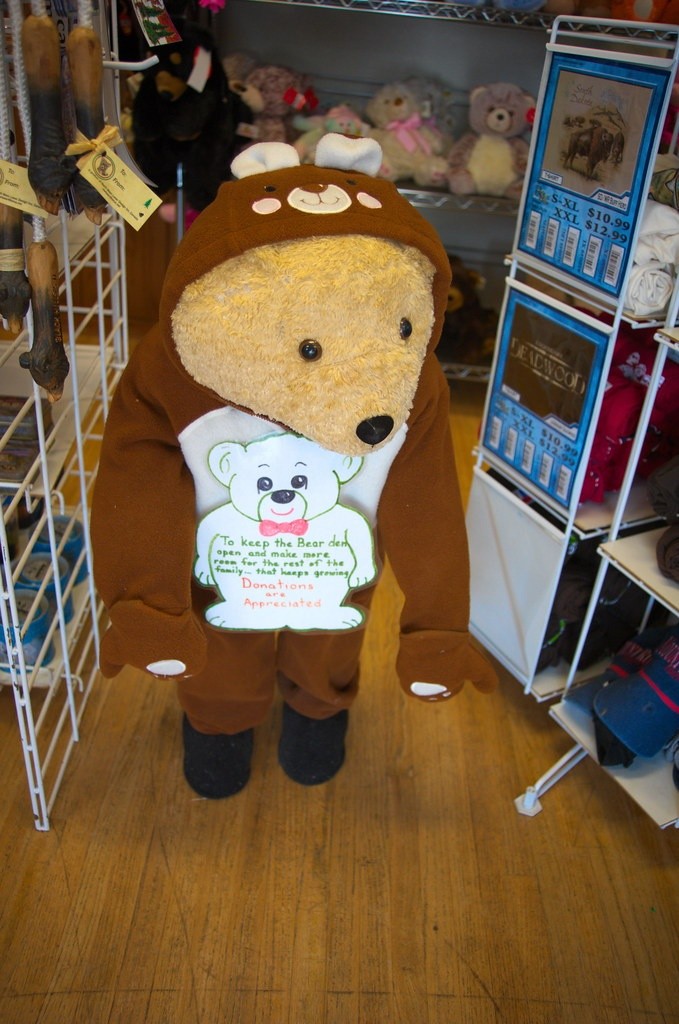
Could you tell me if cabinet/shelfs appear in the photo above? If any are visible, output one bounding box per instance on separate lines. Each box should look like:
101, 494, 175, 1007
465, 16, 679, 830
173, 0, 679, 383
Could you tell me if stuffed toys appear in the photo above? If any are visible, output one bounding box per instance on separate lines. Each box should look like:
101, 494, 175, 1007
89, 133, 470, 802
128, 21, 538, 203
